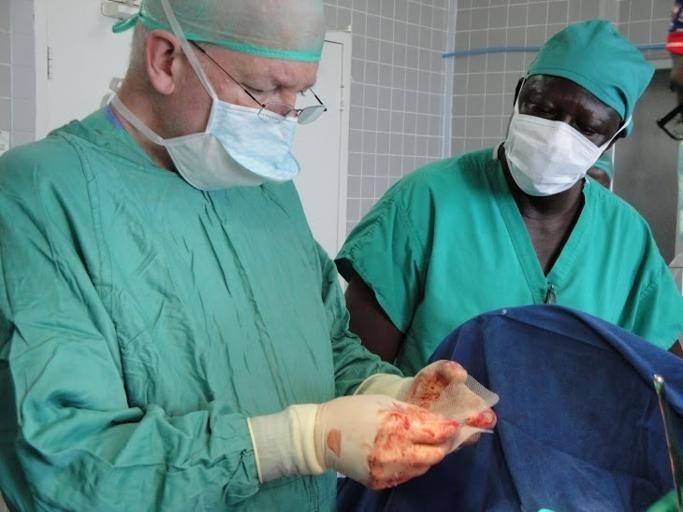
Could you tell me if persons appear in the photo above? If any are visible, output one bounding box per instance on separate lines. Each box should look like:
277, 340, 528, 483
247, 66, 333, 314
331, 18, 683, 376
0, 0, 497, 511
586, 159, 612, 188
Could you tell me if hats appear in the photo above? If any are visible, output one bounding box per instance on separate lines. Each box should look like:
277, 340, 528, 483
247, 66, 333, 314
527, 19, 656, 139
139, 1, 328, 62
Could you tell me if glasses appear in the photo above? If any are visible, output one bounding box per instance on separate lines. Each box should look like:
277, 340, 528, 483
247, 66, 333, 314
188, 41, 328, 124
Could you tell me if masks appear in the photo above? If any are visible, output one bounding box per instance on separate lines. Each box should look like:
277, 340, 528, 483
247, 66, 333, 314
112, 92, 301, 188
503, 107, 600, 198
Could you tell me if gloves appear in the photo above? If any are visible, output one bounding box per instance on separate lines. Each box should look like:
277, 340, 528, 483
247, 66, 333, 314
352, 359, 496, 448
247, 395, 457, 492
